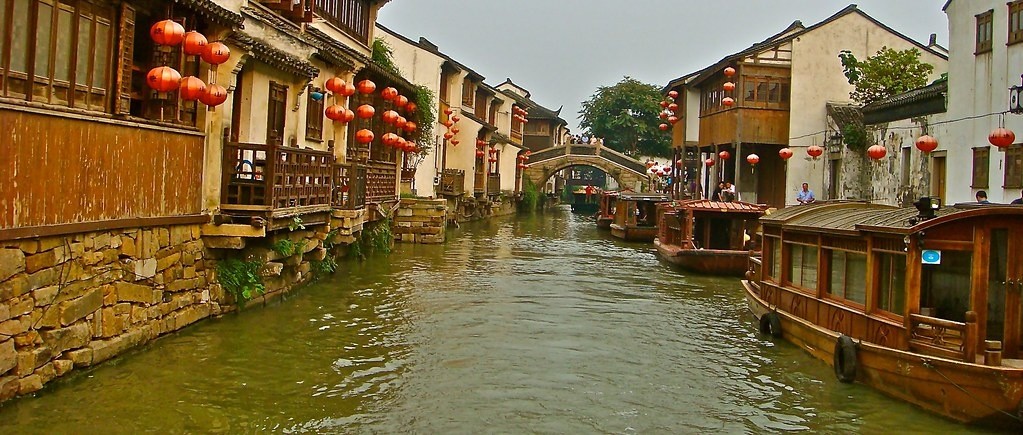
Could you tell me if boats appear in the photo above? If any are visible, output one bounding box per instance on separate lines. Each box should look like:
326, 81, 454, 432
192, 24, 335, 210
652, 200, 771, 276
736, 200, 1023, 435
593, 190, 638, 230
570, 189, 602, 216
608, 193, 672, 244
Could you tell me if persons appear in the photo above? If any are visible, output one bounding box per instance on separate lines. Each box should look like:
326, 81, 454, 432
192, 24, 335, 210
570, 133, 605, 147
975, 189, 989, 203
712, 181, 735, 201
797, 183, 816, 204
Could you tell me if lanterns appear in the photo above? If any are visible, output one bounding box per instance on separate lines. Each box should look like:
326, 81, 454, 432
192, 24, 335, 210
518, 150, 530, 169
647, 158, 682, 176
747, 154, 759, 163
719, 151, 730, 159
916, 135, 938, 153
722, 66, 736, 106
779, 148, 792, 159
706, 159, 715, 166
659, 91, 678, 130
146, 19, 231, 112
444, 108, 460, 146
989, 127, 1015, 151
807, 145, 822, 157
325, 77, 417, 152
867, 144, 886, 160
513, 106, 529, 124
477, 138, 497, 162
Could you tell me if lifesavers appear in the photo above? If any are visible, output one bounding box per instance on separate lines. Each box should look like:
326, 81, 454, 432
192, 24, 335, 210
833, 335, 857, 385
759, 312, 783, 339
